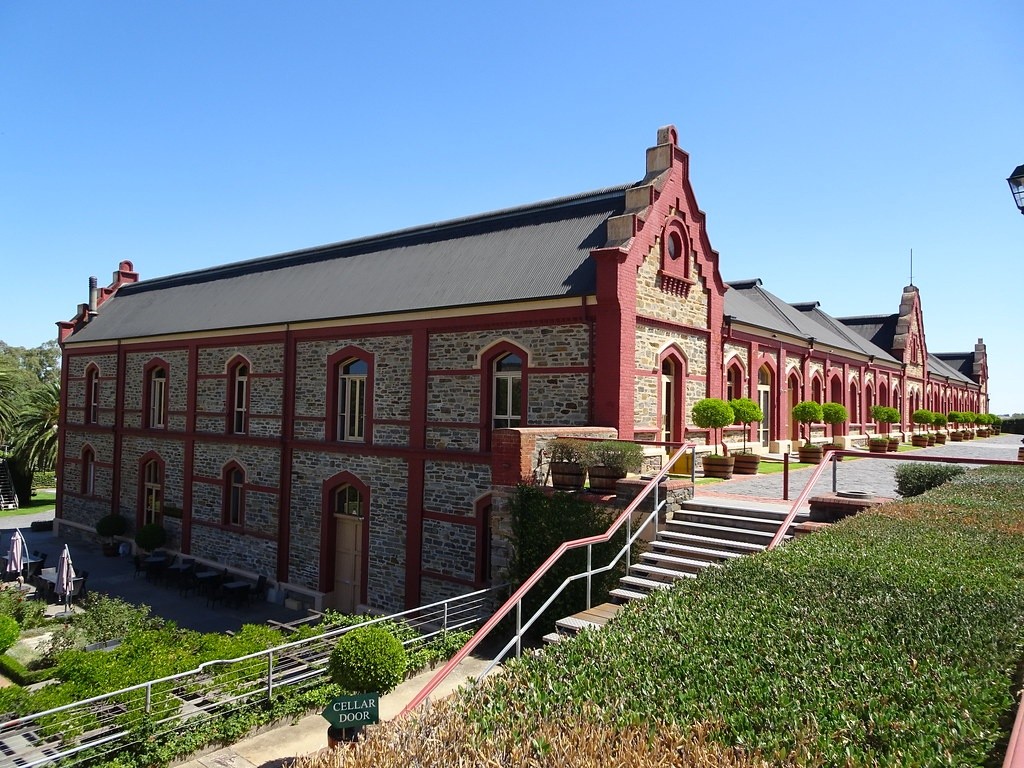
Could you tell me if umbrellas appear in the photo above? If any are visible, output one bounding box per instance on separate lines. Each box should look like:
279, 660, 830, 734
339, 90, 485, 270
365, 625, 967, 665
6, 526, 30, 589
54, 543, 78, 611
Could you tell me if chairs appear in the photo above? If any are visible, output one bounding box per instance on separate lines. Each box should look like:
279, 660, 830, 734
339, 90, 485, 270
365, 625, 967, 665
33, 550, 48, 578
74, 567, 90, 595
41, 567, 56, 591
133, 553, 266, 610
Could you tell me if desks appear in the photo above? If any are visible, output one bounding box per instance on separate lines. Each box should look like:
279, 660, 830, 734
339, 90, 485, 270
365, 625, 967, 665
38, 573, 83, 596
143, 557, 166, 584
194, 571, 219, 597
222, 581, 251, 609
2, 553, 44, 578
167, 563, 192, 590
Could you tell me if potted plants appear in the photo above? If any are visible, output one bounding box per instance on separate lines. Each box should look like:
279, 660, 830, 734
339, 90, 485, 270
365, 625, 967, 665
821, 403, 849, 461
867, 405, 901, 453
691, 398, 735, 479
96, 514, 127, 557
1018, 438, 1024, 461
729, 398, 765, 474
974, 414, 1003, 438
327, 627, 408, 751
947, 411, 977, 442
912, 409, 948, 448
543, 437, 646, 493
792, 401, 824, 464
